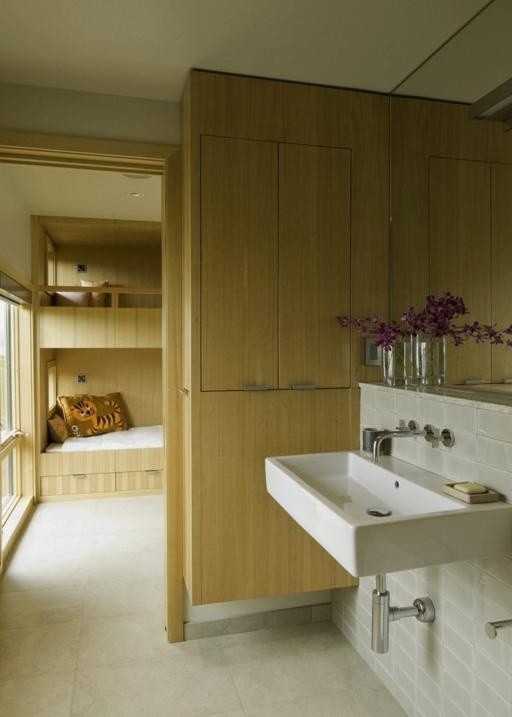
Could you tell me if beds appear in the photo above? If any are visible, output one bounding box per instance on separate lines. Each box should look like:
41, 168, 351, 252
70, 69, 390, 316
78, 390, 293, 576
41, 424, 167, 503
34, 285, 163, 350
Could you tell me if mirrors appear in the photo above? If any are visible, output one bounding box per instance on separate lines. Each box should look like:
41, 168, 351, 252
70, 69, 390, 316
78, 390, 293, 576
387, 0, 511, 398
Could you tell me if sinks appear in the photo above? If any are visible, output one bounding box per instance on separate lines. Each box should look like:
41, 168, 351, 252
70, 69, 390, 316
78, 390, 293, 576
264, 448, 512, 574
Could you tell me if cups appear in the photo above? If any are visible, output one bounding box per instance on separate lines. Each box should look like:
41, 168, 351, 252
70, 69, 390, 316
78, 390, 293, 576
362, 427, 386, 452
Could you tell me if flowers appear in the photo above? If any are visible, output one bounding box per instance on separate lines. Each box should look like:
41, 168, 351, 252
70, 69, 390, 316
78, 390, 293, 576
402, 290, 510, 348
338, 307, 433, 387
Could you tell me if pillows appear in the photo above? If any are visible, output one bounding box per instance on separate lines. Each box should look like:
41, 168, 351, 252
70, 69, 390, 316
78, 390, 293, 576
47, 391, 129, 445
53, 279, 109, 307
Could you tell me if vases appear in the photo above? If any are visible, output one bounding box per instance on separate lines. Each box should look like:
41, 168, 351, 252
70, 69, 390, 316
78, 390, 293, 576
381, 334, 414, 389
414, 334, 446, 387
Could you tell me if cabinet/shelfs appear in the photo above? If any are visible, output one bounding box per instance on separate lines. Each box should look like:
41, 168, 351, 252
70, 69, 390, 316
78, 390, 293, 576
178, 69, 390, 607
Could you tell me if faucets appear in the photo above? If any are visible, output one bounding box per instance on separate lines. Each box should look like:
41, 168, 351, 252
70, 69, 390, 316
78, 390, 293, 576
372, 423, 434, 464
